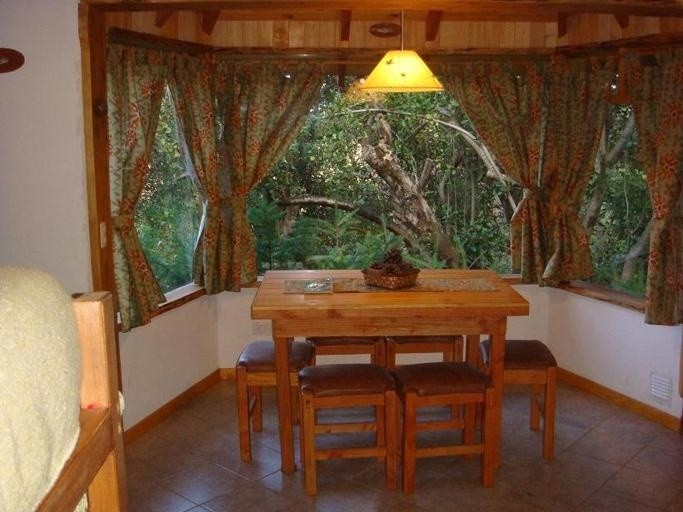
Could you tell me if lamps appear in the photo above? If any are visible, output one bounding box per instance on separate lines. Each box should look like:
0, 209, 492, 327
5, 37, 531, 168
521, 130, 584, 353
356, 10, 445, 93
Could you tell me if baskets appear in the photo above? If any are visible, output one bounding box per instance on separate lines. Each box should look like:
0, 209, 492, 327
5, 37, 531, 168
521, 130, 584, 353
365, 267, 421, 290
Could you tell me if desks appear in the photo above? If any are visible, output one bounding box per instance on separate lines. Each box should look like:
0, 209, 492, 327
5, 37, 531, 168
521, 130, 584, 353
250, 269, 529, 475
